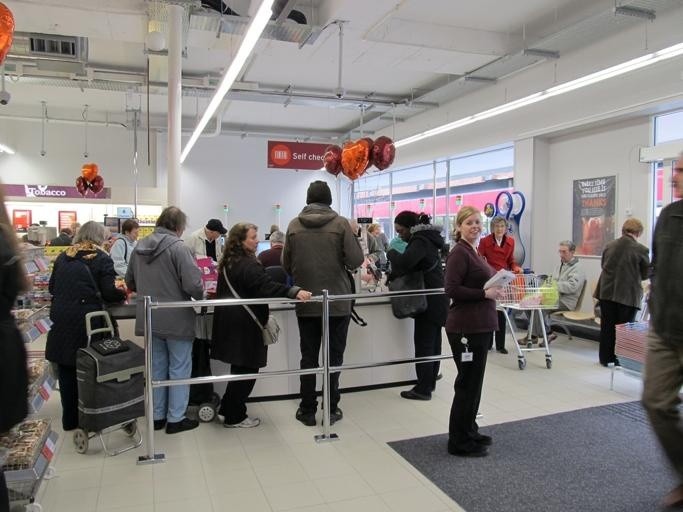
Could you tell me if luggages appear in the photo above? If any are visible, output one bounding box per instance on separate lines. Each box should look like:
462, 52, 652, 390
78, 311, 146, 432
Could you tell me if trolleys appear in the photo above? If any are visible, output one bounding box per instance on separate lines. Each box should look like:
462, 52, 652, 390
71, 311, 146, 457
486, 270, 560, 372
174, 306, 220, 424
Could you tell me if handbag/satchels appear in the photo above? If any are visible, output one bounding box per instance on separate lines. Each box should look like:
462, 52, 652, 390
262, 314, 282, 346
389, 271, 429, 320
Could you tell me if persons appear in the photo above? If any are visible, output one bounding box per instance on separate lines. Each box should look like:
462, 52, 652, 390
0, 180, 29, 512
477, 216, 524, 354
387, 211, 449, 400
110, 219, 141, 281
350, 218, 389, 269
44, 220, 127, 430
210, 222, 311, 428
444, 206, 503, 458
124, 207, 204, 434
186, 219, 225, 261
592, 219, 651, 366
642, 153, 683, 508
283, 182, 364, 426
256, 225, 287, 267
50, 228, 74, 246
518, 239, 584, 347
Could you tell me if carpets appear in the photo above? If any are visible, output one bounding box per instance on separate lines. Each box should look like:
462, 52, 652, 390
387, 400, 682, 511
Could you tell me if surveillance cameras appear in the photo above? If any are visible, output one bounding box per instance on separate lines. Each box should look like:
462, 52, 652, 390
0, 91, 11, 105
83, 152, 89, 158
336, 88, 345, 99
40, 151, 46, 156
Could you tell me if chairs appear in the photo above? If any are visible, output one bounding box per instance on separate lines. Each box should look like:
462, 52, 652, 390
542, 276, 651, 348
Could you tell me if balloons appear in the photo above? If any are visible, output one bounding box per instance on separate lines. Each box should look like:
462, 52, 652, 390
0, 0, 16, 69
322, 136, 396, 182
76, 163, 105, 196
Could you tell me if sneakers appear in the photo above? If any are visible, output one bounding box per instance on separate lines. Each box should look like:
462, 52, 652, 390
154, 418, 167, 431
518, 333, 557, 347
401, 388, 432, 401
165, 417, 200, 434
448, 437, 489, 457
223, 417, 260, 428
497, 346, 508, 354
295, 408, 317, 426
320, 408, 343, 426
600, 357, 622, 368
473, 433, 494, 447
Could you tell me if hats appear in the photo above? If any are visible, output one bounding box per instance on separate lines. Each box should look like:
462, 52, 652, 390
307, 181, 332, 205
206, 219, 227, 234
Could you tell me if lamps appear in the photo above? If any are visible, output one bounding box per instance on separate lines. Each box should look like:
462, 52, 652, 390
179, 0, 276, 163
341, 0, 682, 149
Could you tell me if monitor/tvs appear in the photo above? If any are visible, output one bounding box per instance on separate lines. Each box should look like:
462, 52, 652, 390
255, 240, 270, 256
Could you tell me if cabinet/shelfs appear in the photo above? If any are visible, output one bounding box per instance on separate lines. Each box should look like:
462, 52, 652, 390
134, 214, 161, 240
7, 243, 59, 416
